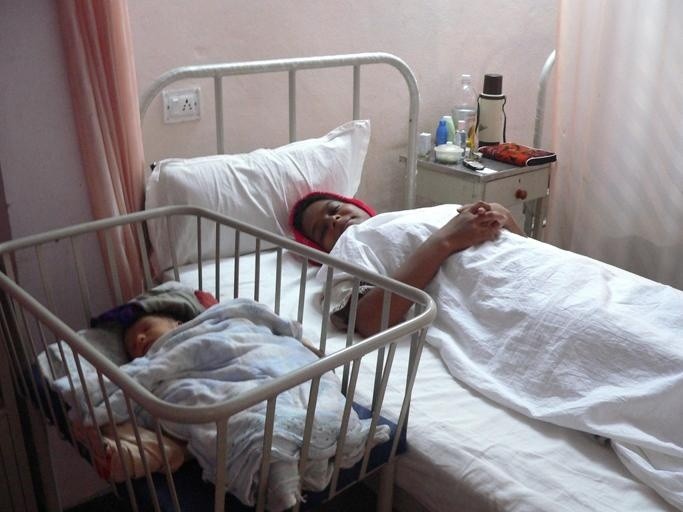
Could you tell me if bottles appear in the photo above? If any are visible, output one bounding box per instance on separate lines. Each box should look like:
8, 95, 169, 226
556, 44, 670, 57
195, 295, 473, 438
454, 119, 467, 159
435, 119, 448, 147
453, 73, 477, 161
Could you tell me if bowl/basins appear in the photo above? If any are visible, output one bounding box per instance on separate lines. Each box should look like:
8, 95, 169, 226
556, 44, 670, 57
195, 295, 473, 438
432, 140, 465, 165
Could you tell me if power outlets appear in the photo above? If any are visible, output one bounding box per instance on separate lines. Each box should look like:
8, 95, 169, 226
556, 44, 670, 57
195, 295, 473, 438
162, 88, 201, 122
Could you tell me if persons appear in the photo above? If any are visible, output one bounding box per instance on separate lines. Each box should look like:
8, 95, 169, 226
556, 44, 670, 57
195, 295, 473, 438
288, 191, 529, 340
116, 301, 198, 362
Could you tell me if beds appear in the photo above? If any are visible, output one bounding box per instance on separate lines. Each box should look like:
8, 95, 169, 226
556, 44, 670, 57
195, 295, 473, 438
136, 52, 683, 512
0, 206, 437, 511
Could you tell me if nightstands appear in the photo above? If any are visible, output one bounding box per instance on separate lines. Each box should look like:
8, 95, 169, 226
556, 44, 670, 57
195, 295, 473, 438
399, 144, 553, 245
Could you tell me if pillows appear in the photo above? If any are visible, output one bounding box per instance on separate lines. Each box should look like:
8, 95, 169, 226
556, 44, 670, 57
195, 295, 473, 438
143, 120, 373, 272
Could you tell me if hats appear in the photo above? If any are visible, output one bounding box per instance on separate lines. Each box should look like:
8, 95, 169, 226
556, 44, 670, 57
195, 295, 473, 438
287, 189, 376, 265
